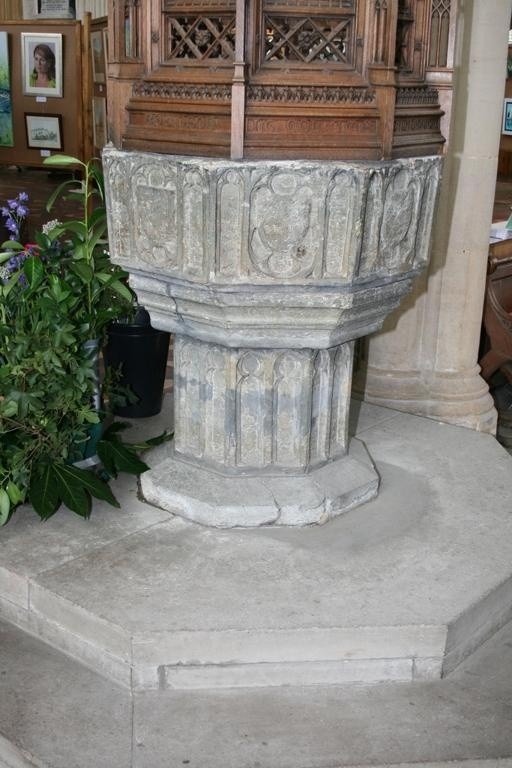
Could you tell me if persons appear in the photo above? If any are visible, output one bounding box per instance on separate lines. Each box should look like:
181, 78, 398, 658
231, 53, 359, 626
29, 43, 56, 87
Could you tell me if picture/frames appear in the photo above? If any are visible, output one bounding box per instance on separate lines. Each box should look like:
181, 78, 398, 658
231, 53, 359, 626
18, 30, 65, 100
23, 110, 66, 154
